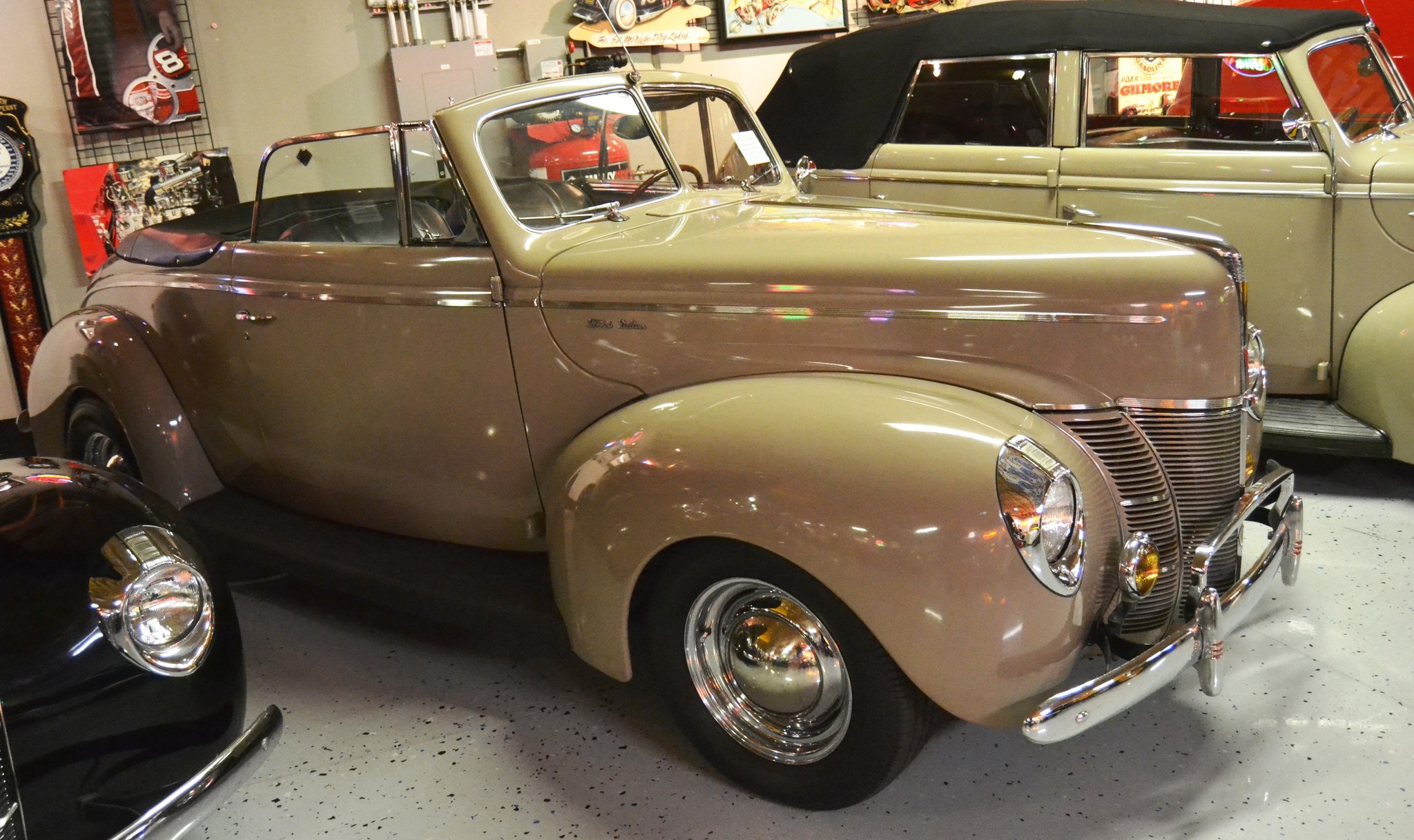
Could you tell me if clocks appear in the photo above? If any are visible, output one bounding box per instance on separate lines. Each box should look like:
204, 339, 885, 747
0, 94, 56, 411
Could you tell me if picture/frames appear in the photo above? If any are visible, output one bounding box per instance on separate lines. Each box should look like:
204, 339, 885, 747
715, 0, 850, 50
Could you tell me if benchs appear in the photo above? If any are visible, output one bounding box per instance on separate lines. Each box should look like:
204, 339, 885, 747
1086, 126, 1201, 150
896, 68, 1055, 146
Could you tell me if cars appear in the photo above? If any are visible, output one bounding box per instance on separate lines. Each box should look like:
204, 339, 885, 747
0, 454, 283, 840
715, 0, 1412, 478
24, 69, 1307, 813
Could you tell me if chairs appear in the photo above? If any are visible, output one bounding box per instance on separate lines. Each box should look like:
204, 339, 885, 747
448, 182, 600, 249
281, 200, 453, 245
